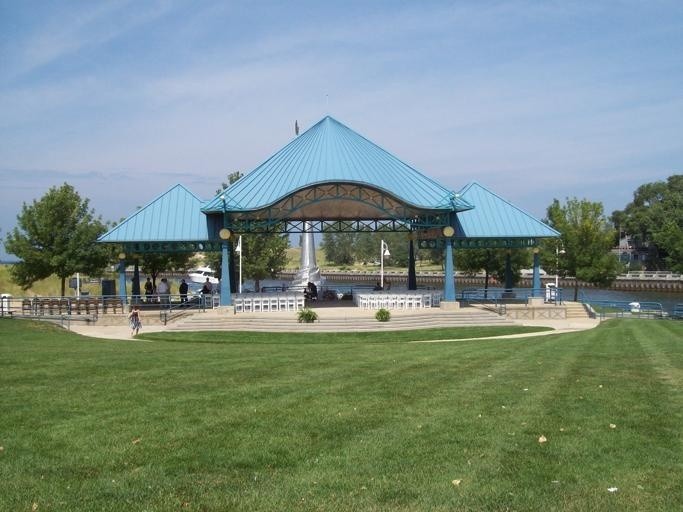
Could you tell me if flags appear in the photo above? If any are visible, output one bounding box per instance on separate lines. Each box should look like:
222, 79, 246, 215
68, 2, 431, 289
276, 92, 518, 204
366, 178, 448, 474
383, 241, 390, 255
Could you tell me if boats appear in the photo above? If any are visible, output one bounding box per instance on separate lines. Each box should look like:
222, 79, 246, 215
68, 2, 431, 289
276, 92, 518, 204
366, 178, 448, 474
188, 265, 219, 286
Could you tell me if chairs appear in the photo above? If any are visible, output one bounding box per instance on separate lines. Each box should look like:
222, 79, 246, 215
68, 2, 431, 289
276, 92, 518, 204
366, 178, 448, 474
351, 288, 445, 310
21, 296, 125, 315
199, 290, 306, 313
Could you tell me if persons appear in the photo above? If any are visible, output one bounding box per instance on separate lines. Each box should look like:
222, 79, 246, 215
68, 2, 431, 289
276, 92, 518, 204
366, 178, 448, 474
131, 277, 220, 308
304, 282, 318, 299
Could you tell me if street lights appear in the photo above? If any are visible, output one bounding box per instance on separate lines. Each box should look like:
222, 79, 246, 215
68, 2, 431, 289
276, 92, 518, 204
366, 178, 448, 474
554, 243, 567, 289
118, 253, 129, 305
379, 239, 391, 287
234, 235, 245, 292
531, 247, 542, 296
443, 226, 458, 302
218, 229, 233, 305
